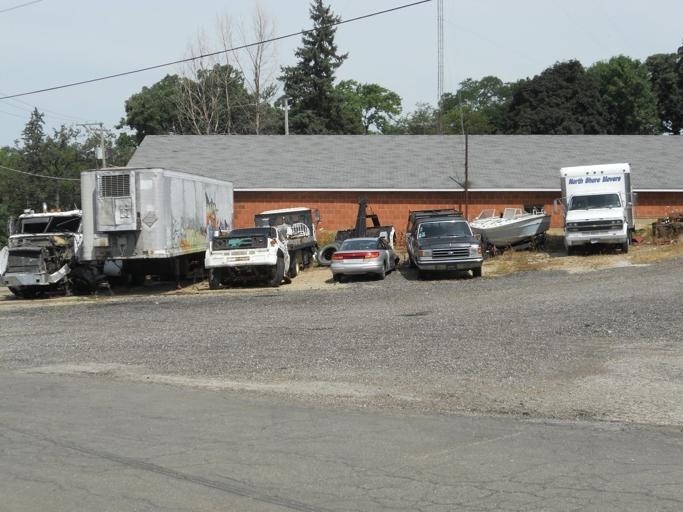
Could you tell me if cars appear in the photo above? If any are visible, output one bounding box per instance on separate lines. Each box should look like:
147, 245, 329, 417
205, 208, 401, 287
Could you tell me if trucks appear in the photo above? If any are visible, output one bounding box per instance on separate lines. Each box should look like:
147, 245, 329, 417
3, 208, 83, 298
554, 162, 638, 254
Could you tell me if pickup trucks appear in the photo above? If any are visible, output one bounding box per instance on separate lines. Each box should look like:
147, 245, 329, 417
406, 208, 484, 280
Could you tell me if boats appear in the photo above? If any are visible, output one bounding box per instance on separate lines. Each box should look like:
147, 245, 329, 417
469, 207, 551, 247
